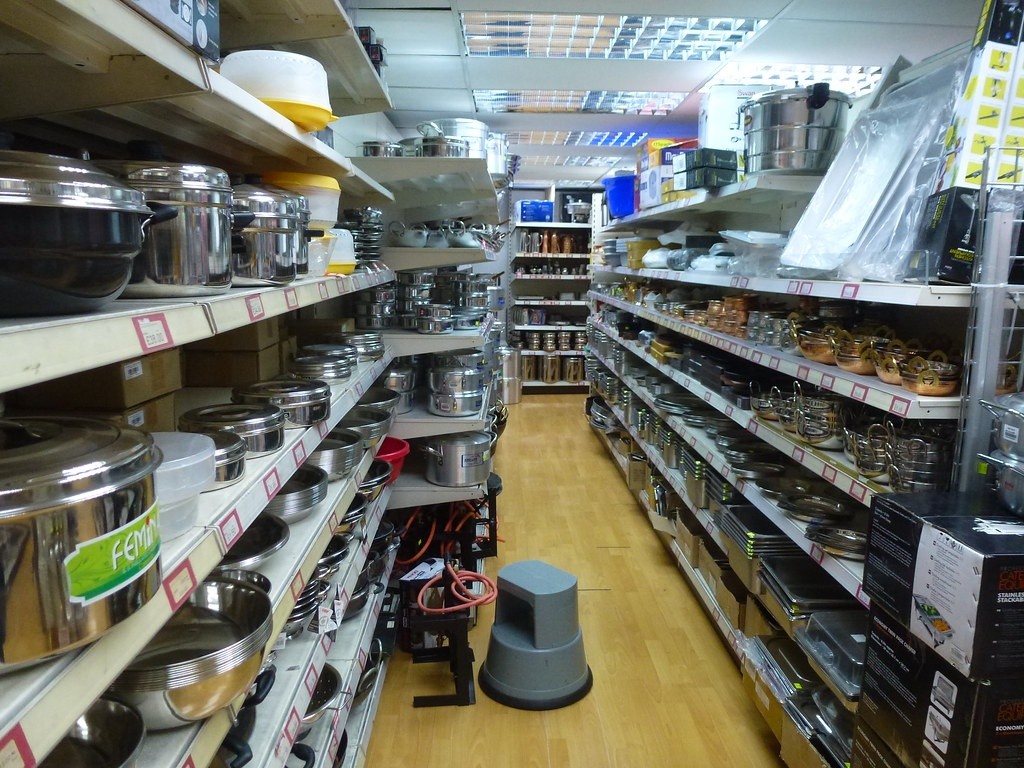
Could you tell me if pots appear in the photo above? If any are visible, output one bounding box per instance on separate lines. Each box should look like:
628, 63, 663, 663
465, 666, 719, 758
739, 81, 853, 174
0, 118, 521, 768
684, 292, 959, 494
976, 393, 1024, 519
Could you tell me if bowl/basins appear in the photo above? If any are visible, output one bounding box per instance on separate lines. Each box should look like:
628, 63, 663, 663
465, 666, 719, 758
585, 356, 872, 700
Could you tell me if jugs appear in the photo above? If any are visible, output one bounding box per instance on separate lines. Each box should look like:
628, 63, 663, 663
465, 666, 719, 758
521, 232, 589, 253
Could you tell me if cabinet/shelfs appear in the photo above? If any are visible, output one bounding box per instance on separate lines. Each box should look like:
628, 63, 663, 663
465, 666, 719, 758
582, 170, 1024, 768
0, 0, 402, 768
345, 155, 501, 635
505, 221, 592, 396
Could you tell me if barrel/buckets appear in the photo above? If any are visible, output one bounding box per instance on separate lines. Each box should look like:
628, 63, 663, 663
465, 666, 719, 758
520, 355, 583, 383
601, 175, 636, 218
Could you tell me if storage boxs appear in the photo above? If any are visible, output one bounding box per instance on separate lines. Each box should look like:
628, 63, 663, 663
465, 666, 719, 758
672, 149, 738, 174
637, 150, 659, 174
904, 0, 1024, 286
514, 199, 553, 222
398, 556, 458, 653
862, 488, 1024, 682
636, 137, 675, 159
640, 167, 662, 210
122, 0, 220, 66
601, 174, 638, 219
850, 711, 904, 768
698, 83, 785, 171
673, 166, 737, 191
857, 598, 1024, 768
649, 149, 696, 182
50, 290, 355, 432
